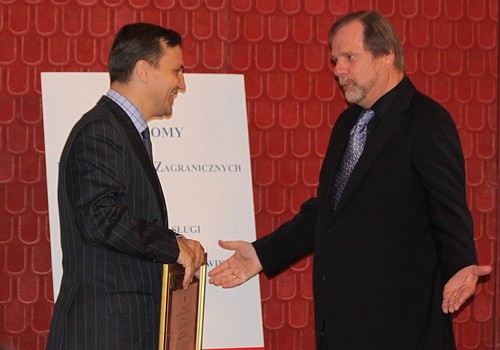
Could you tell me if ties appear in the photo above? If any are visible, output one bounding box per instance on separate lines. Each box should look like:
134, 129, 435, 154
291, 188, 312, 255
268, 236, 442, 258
329, 107, 376, 212
140, 124, 154, 170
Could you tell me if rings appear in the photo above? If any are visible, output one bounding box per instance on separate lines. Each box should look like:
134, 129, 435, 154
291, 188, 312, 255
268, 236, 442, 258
232, 273, 236, 279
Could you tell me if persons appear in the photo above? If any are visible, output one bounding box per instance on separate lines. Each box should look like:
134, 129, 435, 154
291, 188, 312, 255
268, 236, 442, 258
47, 22, 206, 350
209, 9, 492, 350
